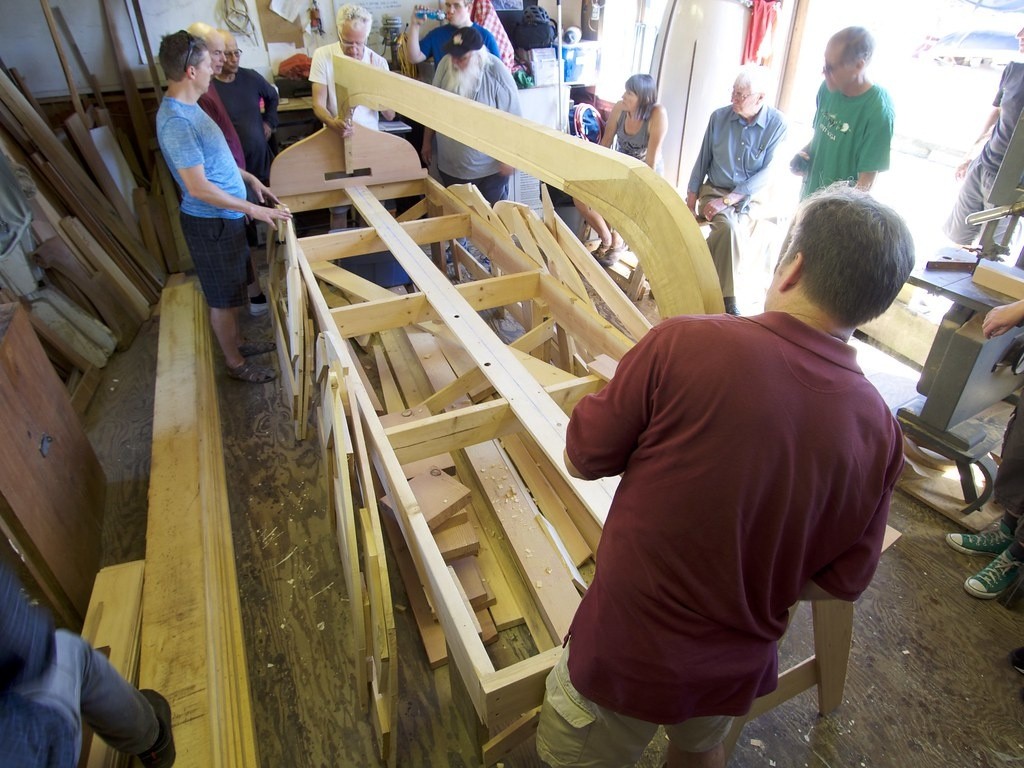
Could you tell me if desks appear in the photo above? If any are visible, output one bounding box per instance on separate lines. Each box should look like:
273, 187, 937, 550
260, 96, 318, 156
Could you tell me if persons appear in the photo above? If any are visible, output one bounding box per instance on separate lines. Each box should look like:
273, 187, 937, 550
945, 299, 1024, 600
686, 65, 789, 316
573, 72, 670, 266
1008, 646, 1024, 675
536, 185, 915, 768
307, 0, 522, 267
788, 27, 893, 201
155, 23, 293, 384
946, 28, 1024, 260
0, 564, 177, 768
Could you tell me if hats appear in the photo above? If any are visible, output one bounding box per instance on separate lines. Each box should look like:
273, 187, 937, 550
444, 27, 483, 56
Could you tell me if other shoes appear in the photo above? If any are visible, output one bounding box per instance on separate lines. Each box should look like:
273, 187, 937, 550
250, 296, 269, 317
138, 688, 176, 767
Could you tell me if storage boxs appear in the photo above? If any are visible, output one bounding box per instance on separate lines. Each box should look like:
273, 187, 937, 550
274, 76, 312, 98
530, 39, 601, 87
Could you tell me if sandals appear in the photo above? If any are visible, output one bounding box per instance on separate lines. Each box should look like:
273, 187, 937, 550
593, 242, 623, 265
226, 360, 277, 382
239, 341, 276, 355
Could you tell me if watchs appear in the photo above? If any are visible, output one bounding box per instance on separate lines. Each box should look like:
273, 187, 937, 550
724, 196, 731, 206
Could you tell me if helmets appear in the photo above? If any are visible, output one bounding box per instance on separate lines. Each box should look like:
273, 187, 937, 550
522, 4, 550, 25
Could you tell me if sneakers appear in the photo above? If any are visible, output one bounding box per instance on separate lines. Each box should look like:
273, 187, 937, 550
945, 517, 1015, 558
964, 555, 1023, 599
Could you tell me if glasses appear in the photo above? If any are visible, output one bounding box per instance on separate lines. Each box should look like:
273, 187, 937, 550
225, 49, 243, 57
180, 29, 195, 73
825, 59, 858, 74
339, 31, 367, 48
731, 89, 759, 100
